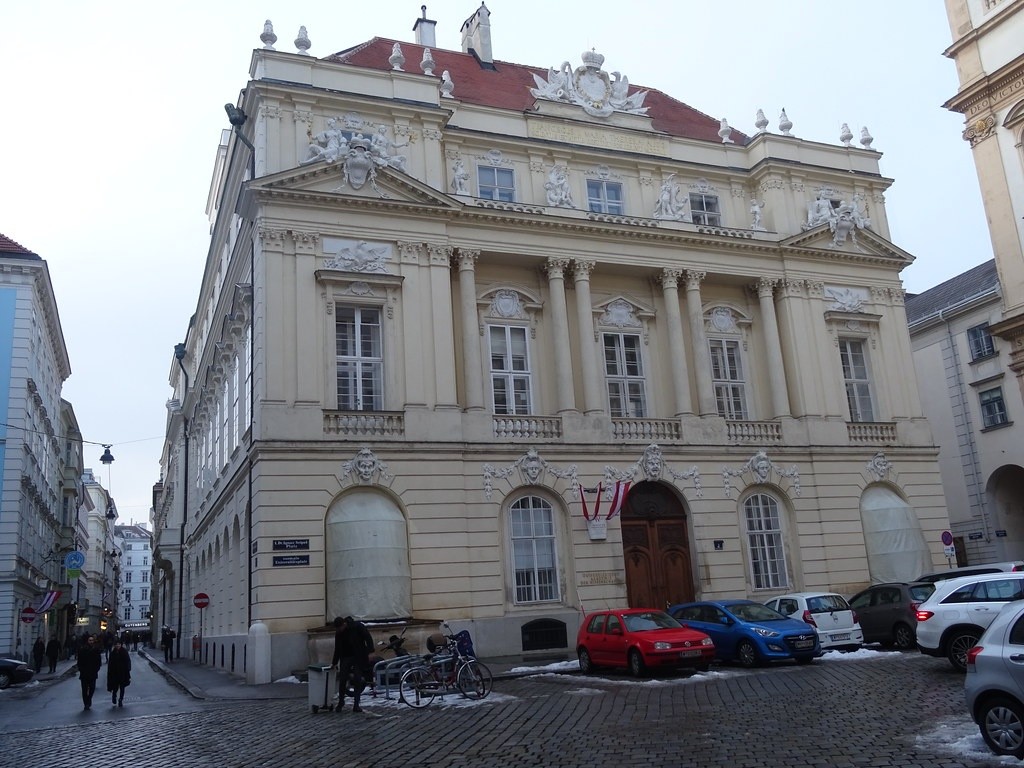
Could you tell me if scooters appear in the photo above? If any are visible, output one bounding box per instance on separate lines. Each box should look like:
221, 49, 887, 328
339, 626, 420, 698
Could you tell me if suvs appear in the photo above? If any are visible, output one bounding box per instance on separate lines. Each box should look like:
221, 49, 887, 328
963, 598, 1024, 757
915, 571, 1024, 676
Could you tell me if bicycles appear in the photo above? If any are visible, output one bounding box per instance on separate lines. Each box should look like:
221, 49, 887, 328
399, 622, 493, 708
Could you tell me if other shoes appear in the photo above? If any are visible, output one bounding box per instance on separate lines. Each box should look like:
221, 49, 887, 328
48, 668, 56, 673
166, 660, 174, 665
337, 699, 362, 714
36, 668, 40, 673
83, 698, 92, 711
111, 695, 123, 706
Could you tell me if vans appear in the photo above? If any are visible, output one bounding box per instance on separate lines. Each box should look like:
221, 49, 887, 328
912, 561, 1024, 599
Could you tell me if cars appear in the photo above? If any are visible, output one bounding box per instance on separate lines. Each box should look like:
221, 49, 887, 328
574, 607, 715, 678
666, 599, 823, 670
846, 581, 936, 650
756, 592, 864, 655
0, 658, 35, 690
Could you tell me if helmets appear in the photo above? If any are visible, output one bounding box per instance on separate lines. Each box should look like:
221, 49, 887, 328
427, 633, 447, 653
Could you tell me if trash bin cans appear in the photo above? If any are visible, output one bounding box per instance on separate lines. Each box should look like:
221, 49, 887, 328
308, 664, 337, 714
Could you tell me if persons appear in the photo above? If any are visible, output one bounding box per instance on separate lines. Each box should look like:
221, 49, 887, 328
526, 450, 540, 478
107, 641, 132, 711
298, 117, 408, 174
750, 198, 767, 225
33, 636, 45, 674
544, 166, 576, 208
331, 616, 375, 712
64, 630, 153, 661
655, 174, 686, 216
77, 636, 102, 712
754, 455, 770, 477
873, 454, 888, 476
161, 628, 176, 663
45, 635, 61, 674
352, 450, 376, 481
803, 190, 872, 229
451, 161, 471, 192
643, 448, 661, 476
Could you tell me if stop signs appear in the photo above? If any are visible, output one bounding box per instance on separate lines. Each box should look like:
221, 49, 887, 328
193, 593, 209, 609
21, 607, 36, 624
